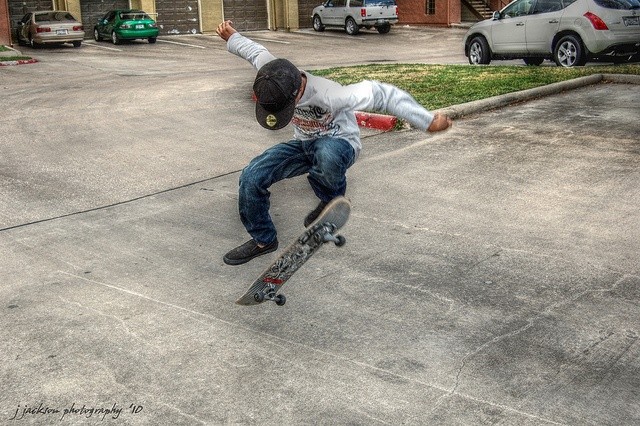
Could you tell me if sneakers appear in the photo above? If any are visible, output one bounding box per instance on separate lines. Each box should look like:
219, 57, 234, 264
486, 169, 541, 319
223, 238, 278, 265
304, 200, 326, 228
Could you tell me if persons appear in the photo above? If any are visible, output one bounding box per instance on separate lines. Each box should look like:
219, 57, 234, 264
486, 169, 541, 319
215, 19, 452, 265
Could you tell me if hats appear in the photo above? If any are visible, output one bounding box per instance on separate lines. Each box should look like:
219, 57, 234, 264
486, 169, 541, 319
253, 59, 301, 130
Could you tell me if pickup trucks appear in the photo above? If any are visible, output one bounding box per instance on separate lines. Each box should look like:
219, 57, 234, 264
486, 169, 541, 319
310, 0, 399, 34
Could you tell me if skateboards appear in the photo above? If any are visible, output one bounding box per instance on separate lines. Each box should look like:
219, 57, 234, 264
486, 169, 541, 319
236, 195, 351, 307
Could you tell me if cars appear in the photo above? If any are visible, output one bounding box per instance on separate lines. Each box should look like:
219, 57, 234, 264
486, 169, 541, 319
463, 0, 639, 68
17, 10, 85, 48
94, 8, 160, 44
